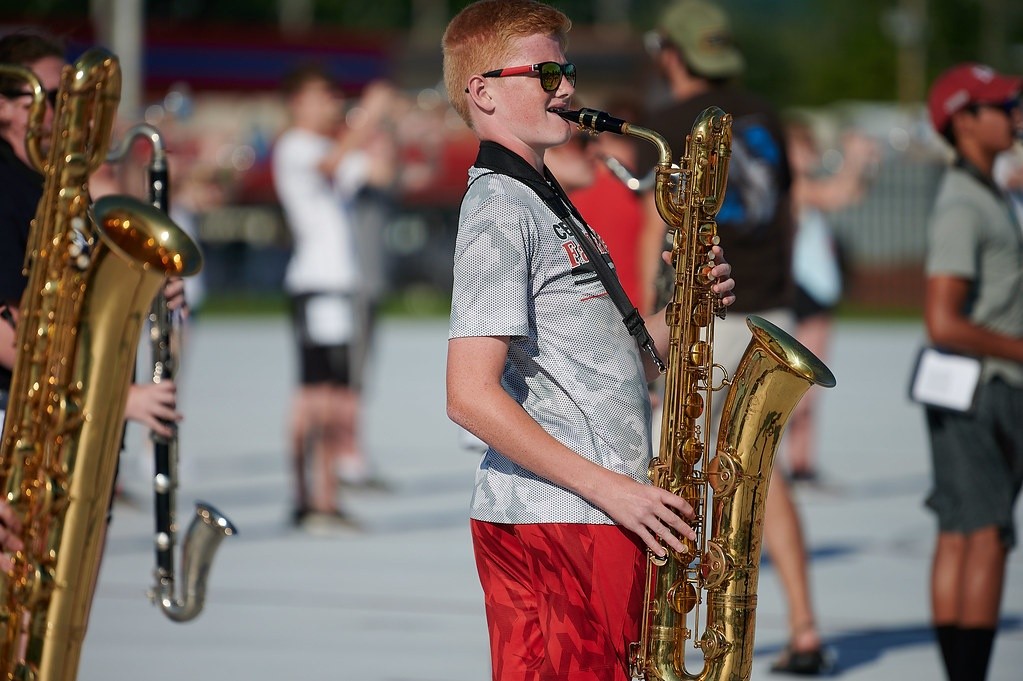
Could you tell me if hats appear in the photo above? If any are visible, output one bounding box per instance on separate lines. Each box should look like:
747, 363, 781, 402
930, 62, 1023, 133
664, 2, 745, 74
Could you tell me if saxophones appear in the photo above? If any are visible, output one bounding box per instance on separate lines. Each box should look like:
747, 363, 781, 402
556, 103, 838, 681
0, 45, 204, 681
101, 122, 239, 624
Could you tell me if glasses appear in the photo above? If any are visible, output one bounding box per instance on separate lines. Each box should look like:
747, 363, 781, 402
0, 89, 57, 109
465, 61, 576, 94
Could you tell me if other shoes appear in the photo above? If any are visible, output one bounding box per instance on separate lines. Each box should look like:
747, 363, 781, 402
771, 646, 824, 676
298, 511, 366, 541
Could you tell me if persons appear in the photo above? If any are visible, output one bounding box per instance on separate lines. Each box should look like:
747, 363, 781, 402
268, 73, 448, 536
543, 1, 870, 681
908, 64, 1023, 681
0, 35, 194, 521
439, 1, 737, 680
